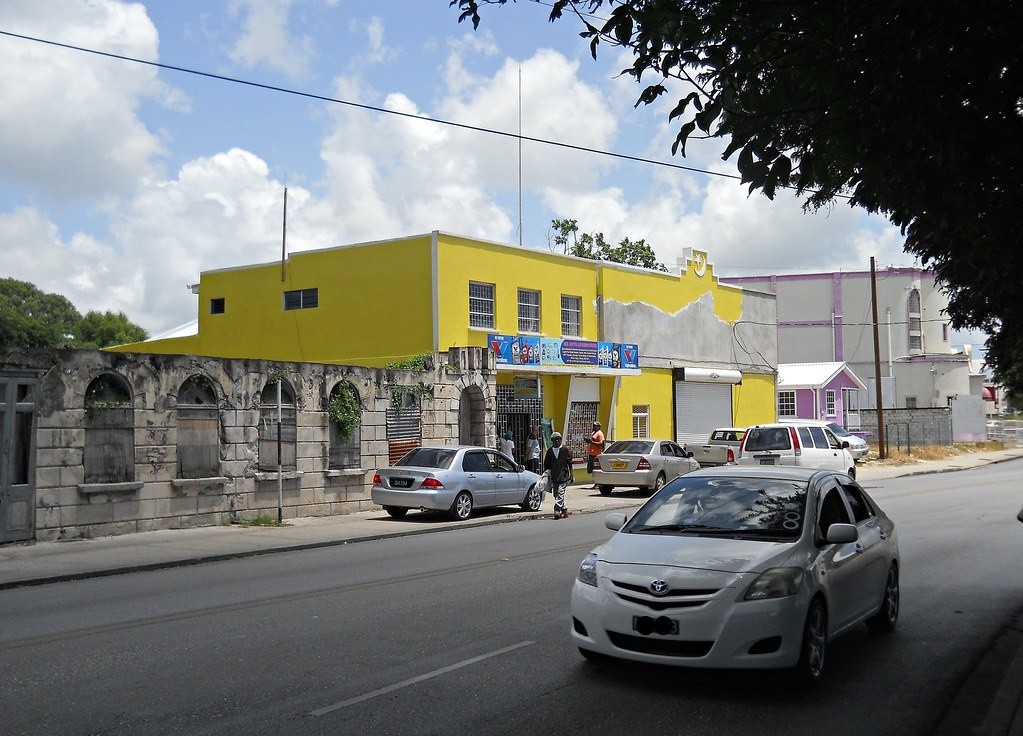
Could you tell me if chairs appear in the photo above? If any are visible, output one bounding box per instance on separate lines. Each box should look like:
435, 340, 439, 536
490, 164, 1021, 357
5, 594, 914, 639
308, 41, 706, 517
729, 435, 736, 441
777, 436, 788, 448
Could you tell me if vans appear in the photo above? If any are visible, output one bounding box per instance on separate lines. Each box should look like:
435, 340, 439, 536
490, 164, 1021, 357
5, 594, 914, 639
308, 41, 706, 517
775, 419, 871, 463
734, 423, 856, 484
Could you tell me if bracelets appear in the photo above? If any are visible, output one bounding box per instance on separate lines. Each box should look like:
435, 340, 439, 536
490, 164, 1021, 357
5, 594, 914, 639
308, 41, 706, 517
570, 474, 572, 476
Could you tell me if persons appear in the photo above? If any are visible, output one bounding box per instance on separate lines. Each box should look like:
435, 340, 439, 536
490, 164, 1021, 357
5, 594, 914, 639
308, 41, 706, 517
585, 421, 606, 488
544, 433, 575, 519
524, 426, 541, 473
496, 427, 517, 466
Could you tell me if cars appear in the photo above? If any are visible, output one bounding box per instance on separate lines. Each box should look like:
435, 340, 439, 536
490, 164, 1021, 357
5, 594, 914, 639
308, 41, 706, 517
372, 444, 546, 521
593, 437, 703, 496
568, 465, 905, 690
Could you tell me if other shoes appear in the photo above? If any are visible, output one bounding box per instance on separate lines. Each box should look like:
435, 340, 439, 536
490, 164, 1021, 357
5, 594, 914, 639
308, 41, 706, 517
553, 506, 561, 519
561, 508, 569, 518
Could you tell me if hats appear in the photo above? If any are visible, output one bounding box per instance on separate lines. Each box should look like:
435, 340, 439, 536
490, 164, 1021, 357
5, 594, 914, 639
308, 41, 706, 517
593, 421, 602, 426
550, 432, 562, 440
506, 431, 513, 437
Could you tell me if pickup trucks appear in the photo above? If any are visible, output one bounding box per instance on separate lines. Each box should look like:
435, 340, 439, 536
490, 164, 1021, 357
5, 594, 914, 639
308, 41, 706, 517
682, 426, 749, 468
